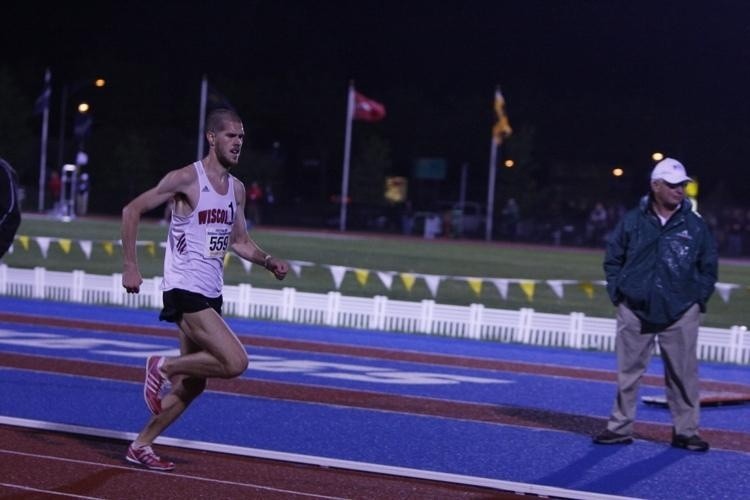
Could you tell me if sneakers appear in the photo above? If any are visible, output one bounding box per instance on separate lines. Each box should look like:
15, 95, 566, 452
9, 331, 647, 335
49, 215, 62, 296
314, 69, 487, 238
592, 429, 632, 444
143, 355, 169, 416
125, 442, 176, 470
671, 435, 709, 451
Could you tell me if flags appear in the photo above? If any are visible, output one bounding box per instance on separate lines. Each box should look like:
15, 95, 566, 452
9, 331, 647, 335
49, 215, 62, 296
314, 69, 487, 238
491, 92, 514, 146
352, 89, 388, 124
206, 82, 234, 113
30, 79, 54, 116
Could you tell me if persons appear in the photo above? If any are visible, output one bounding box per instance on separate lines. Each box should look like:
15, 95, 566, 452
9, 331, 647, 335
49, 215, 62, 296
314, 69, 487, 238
0, 158, 23, 261
594, 156, 719, 450
120, 107, 288, 471
50, 169, 60, 213
245, 177, 750, 262
76, 173, 89, 216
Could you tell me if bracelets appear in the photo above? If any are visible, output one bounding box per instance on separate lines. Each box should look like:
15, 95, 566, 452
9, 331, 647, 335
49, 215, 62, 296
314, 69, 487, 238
264, 254, 272, 269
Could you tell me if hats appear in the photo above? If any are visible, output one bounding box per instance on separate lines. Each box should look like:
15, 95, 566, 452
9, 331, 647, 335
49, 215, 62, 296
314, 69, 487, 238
650, 158, 693, 185
